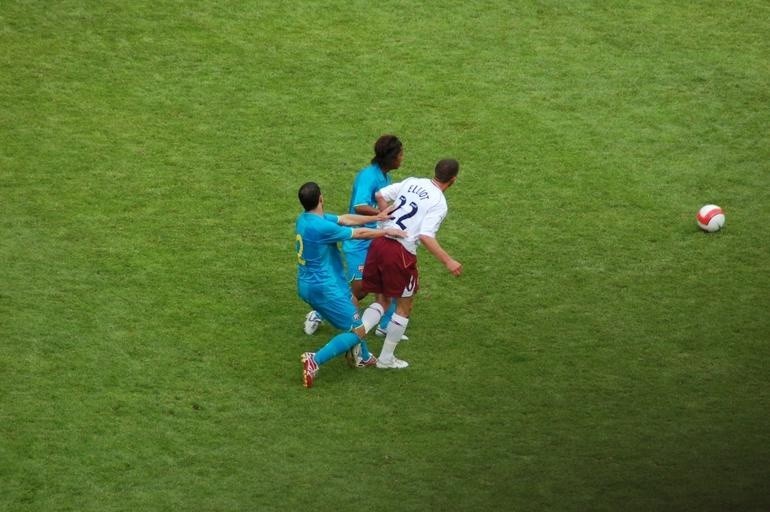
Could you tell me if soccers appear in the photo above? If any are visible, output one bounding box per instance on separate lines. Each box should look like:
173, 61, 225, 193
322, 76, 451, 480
697, 203, 725, 232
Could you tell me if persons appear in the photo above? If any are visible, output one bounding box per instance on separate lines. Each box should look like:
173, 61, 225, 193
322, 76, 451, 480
294, 180, 409, 386
303, 134, 410, 341
352, 155, 465, 370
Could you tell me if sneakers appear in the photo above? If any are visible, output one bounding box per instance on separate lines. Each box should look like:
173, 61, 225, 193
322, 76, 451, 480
376, 357, 408, 368
346, 344, 377, 368
301, 352, 319, 388
375, 325, 409, 340
303, 310, 319, 335
